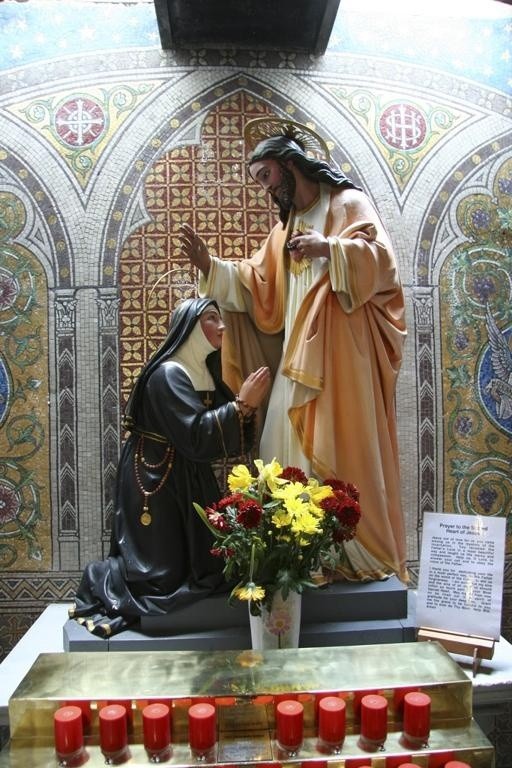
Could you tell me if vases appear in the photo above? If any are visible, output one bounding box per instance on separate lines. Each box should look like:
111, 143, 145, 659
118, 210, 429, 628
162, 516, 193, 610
248, 587, 303, 651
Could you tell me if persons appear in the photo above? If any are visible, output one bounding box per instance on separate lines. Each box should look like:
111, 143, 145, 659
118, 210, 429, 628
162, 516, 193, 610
66, 297, 272, 637
175, 135, 415, 583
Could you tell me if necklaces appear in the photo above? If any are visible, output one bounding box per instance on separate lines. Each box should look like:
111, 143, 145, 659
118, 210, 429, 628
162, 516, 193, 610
232, 405, 260, 478
176, 354, 214, 410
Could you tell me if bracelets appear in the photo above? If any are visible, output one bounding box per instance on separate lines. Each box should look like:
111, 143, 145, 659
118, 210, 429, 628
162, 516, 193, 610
235, 395, 256, 412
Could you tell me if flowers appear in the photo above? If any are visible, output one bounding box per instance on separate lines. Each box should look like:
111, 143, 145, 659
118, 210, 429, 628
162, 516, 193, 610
192, 456, 362, 616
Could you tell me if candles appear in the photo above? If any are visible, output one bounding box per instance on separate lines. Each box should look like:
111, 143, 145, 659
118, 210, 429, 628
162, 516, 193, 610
275, 690, 474, 768
54, 702, 216, 751
